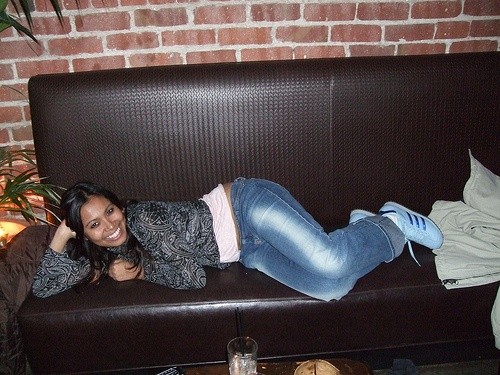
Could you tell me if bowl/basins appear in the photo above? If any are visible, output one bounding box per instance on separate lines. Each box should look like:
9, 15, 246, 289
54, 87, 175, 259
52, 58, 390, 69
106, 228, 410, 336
294, 359, 341, 375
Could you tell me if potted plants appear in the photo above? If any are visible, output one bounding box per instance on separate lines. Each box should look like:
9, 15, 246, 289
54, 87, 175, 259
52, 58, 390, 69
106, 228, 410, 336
0, 144, 65, 248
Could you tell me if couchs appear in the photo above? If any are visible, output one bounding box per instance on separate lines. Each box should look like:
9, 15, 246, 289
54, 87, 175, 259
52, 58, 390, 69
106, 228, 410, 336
17, 51, 500, 375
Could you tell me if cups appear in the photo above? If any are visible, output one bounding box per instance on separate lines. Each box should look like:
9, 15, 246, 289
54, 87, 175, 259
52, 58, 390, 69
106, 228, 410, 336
227, 337, 258, 375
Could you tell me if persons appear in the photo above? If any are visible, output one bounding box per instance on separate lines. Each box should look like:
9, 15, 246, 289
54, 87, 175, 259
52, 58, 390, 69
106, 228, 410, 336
31, 181, 443, 302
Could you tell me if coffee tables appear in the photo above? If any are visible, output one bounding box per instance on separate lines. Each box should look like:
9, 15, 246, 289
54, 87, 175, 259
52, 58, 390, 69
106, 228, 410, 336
181, 359, 369, 375
